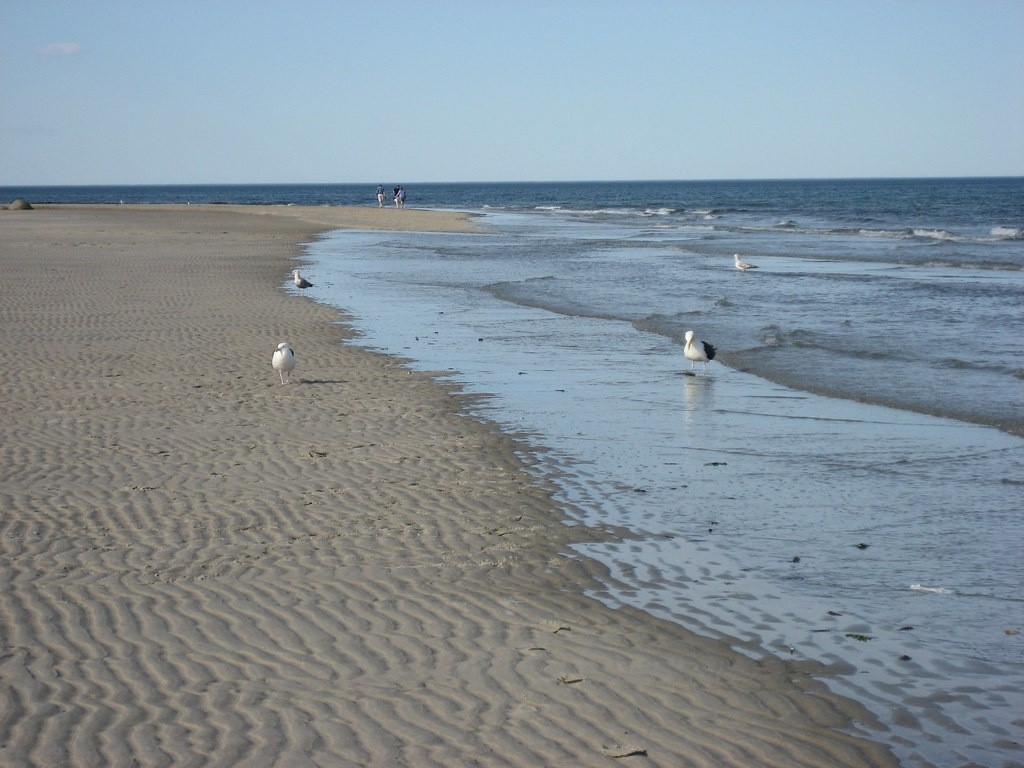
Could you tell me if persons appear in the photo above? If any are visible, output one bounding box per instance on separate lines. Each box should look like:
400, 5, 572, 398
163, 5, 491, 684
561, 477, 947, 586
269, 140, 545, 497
376, 184, 387, 208
392, 185, 407, 209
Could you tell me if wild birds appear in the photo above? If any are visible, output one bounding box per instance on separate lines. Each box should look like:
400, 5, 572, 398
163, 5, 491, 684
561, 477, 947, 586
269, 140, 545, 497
270, 341, 298, 384
684, 329, 720, 375
733, 253, 760, 273
290, 269, 316, 297
